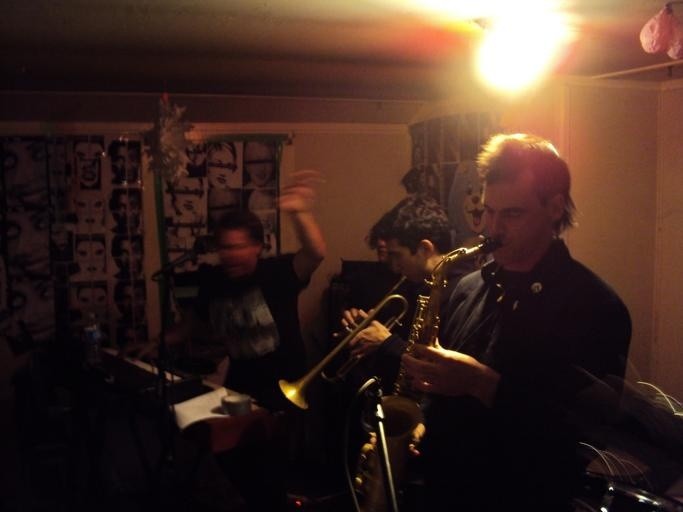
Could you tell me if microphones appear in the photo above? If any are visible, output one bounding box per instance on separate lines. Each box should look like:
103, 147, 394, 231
152, 235, 219, 279
363, 376, 383, 399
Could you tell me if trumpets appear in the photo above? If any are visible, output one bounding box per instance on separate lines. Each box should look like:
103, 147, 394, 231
278, 275, 409, 409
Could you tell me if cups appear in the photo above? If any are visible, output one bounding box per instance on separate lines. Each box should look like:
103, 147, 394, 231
221, 394, 252, 417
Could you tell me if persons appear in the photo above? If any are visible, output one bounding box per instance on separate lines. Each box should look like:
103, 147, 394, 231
0, 132, 275, 343
404, 144, 435, 194
341, 209, 412, 318
352, 128, 632, 511
113, 167, 328, 406
330, 195, 454, 377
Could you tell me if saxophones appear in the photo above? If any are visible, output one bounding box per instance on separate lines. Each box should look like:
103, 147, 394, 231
357, 238, 504, 511
